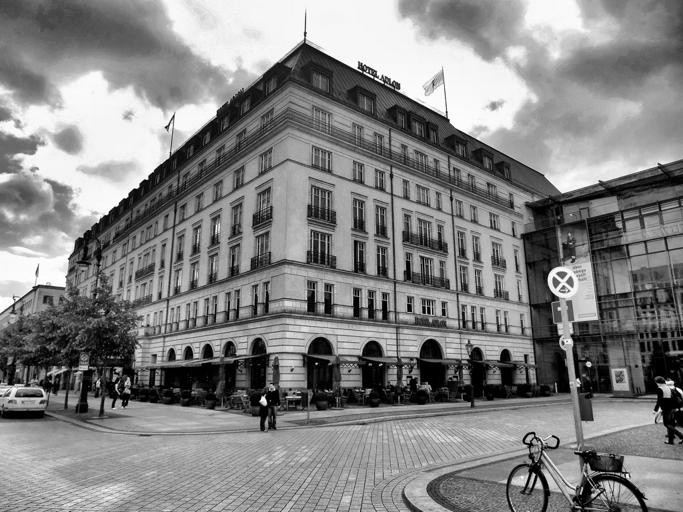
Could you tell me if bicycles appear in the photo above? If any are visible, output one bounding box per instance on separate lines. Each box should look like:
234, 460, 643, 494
506, 429, 651, 512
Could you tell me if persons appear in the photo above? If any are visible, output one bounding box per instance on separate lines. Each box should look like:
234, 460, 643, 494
265, 382, 281, 431
120, 376, 132, 409
111, 377, 121, 410
29, 373, 61, 396
376, 376, 433, 405
651, 375, 682, 444
92, 377, 102, 398
257, 387, 267, 432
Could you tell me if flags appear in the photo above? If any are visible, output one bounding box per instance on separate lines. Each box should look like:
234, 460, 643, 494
164, 111, 175, 132
34, 263, 39, 279
419, 65, 445, 96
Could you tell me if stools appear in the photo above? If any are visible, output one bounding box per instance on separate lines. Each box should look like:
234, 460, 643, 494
277, 401, 287, 411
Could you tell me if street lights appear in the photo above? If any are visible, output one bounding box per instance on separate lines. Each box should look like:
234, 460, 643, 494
74, 231, 102, 414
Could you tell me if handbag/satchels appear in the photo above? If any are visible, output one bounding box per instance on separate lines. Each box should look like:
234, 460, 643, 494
260, 395, 267, 406
123, 387, 130, 394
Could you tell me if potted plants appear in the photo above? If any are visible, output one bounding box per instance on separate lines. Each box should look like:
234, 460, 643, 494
315, 393, 329, 410
414, 390, 429, 405
368, 391, 381, 407
161, 389, 192, 407
250, 393, 262, 415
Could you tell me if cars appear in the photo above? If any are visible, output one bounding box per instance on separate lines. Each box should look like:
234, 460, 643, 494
0, 385, 48, 418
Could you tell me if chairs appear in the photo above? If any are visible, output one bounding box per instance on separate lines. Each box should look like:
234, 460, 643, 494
441, 387, 450, 400
214, 387, 252, 414
362, 388, 372, 405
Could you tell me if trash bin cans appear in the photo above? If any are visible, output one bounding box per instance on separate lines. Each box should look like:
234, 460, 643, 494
579, 392, 594, 421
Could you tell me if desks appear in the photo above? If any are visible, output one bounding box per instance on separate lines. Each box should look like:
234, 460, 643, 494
285, 395, 302, 410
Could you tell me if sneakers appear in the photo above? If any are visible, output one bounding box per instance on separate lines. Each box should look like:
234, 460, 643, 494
665, 435, 683, 444
112, 406, 125, 409
261, 425, 276, 431
